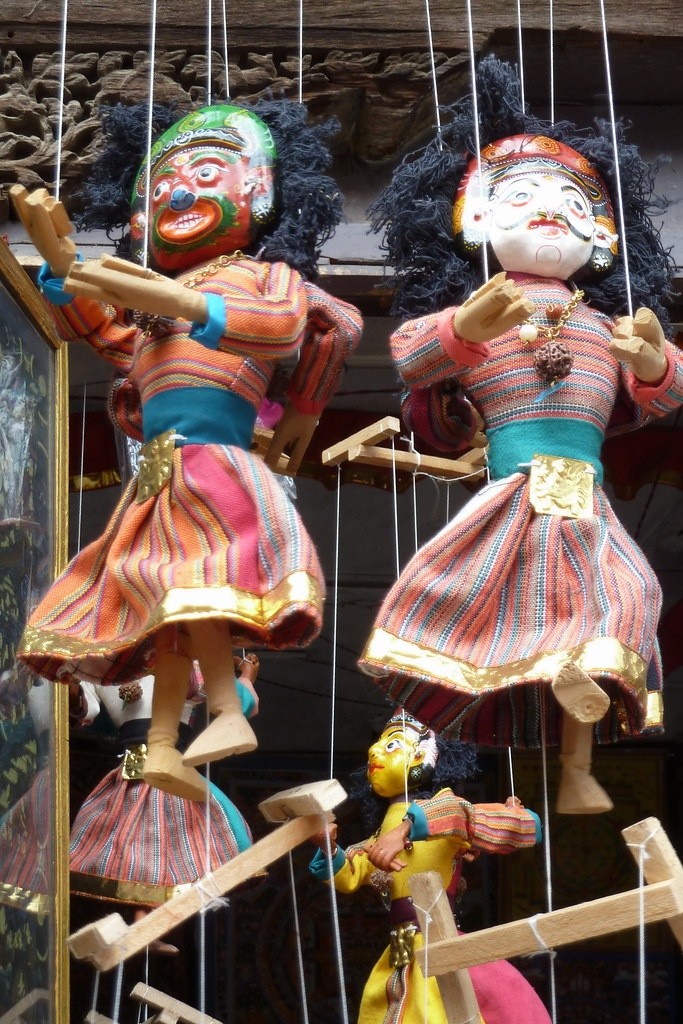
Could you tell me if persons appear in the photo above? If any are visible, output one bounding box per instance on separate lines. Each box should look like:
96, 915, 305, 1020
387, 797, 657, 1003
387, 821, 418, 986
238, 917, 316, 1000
374, 62, 683, 815
310, 707, 558, 1023
70, 649, 269, 953
1, 652, 103, 895
11, 92, 365, 801
